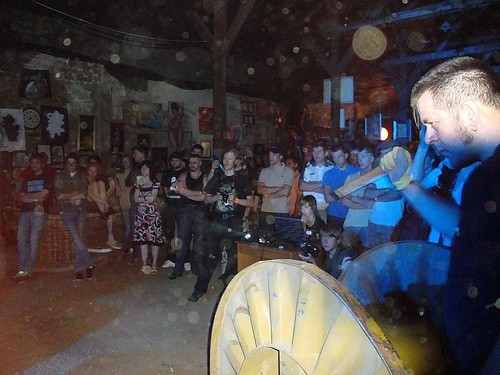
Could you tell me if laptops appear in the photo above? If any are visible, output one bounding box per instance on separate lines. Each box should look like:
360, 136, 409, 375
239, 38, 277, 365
274, 217, 307, 242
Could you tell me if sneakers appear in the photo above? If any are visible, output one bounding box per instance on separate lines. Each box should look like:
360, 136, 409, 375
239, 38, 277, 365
106, 239, 122, 250
184, 262, 191, 271
162, 260, 175, 267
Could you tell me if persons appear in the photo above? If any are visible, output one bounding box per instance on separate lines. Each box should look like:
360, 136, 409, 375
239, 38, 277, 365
300, 195, 326, 246
299, 223, 352, 282
410, 56, 500, 375
11, 154, 53, 278
341, 229, 371, 259
56, 152, 96, 282
187, 148, 255, 305
134, 161, 167, 276
0, 139, 484, 289
171, 155, 209, 278
257, 148, 294, 234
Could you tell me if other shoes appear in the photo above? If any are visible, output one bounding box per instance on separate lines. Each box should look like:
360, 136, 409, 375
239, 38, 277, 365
151, 267, 157, 272
170, 272, 182, 279
76, 271, 84, 281
192, 270, 199, 275
141, 267, 148, 272
12, 271, 31, 279
86, 265, 95, 279
189, 289, 206, 302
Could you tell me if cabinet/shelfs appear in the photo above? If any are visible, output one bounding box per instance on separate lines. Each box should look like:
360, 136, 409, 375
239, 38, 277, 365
236, 241, 323, 273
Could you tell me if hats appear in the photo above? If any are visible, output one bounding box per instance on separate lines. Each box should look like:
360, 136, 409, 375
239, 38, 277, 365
269, 146, 282, 153
172, 154, 181, 159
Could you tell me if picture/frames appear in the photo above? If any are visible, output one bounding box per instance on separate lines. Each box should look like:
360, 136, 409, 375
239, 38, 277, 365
200, 139, 212, 159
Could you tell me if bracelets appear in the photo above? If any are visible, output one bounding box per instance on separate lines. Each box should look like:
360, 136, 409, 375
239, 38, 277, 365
144, 195, 147, 202
200, 190, 203, 195
34, 197, 38, 203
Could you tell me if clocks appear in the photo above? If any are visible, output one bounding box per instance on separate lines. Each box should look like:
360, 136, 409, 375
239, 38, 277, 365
22, 106, 42, 130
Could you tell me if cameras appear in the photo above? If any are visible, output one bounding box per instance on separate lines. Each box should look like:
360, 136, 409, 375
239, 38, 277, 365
299, 243, 320, 258
